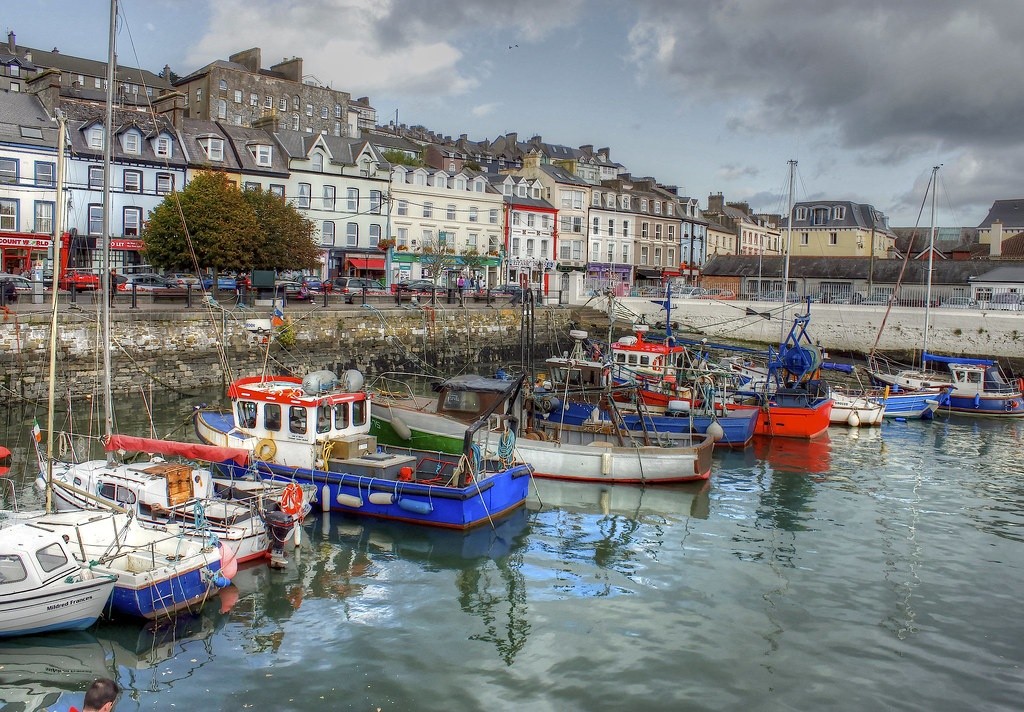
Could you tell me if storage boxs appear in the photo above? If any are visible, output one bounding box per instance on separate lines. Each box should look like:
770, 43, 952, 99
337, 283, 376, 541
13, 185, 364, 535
329, 433, 378, 460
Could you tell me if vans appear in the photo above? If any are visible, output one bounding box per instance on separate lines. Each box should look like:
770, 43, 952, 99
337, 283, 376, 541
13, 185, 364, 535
331, 276, 389, 304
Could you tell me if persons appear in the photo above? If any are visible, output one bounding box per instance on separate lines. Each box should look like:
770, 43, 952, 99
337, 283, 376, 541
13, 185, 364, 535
67, 678, 119, 712
458, 276, 483, 288
300, 282, 313, 302
235, 274, 248, 303
110, 270, 117, 307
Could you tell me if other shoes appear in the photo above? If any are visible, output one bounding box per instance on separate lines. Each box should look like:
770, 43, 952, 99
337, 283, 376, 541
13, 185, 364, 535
111, 305, 115, 308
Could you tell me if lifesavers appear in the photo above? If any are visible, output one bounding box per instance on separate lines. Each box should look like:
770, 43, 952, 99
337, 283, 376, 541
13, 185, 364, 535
282, 482, 303, 514
602, 369, 611, 397
1017, 378, 1023, 390
883, 385, 889, 399
653, 356, 662, 372
274, 388, 304, 398
256, 439, 277, 461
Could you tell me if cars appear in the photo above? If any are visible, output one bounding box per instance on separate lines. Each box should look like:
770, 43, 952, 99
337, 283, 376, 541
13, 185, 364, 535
20, 268, 54, 289
923, 294, 945, 308
699, 288, 736, 300
116, 272, 183, 291
859, 293, 901, 306
803, 291, 839, 304
322, 279, 333, 293
273, 280, 315, 304
159, 272, 202, 291
829, 292, 866, 305
676, 287, 707, 299
395, 279, 448, 302
199, 272, 237, 293
234, 273, 259, 292
0, 273, 49, 291
57, 270, 101, 292
630, 285, 660, 298
295, 276, 325, 293
983, 292, 1024, 311
938, 297, 980, 310
750, 289, 800, 303
490, 284, 532, 306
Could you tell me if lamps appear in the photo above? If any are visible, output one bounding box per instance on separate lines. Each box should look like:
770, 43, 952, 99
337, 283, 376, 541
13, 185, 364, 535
416, 246, 420, 252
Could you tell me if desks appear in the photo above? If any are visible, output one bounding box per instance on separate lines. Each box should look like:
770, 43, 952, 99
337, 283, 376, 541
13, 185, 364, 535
327, 452, 417, 483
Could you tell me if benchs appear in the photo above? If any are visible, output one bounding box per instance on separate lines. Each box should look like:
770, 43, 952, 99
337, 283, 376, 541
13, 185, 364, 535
286, 290, 314, 304
474, 292, 496, 303
395, 295, 421, 303
126, 552, 176, 574
154, 288, 188, 304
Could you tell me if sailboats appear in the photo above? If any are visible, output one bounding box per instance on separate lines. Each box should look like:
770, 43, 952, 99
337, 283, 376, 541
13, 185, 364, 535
0, 0, 1024, 643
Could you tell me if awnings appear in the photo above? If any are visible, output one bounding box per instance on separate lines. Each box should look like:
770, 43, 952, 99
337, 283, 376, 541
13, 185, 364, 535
638, 273, 663, 279
349, 259, 385, 269
